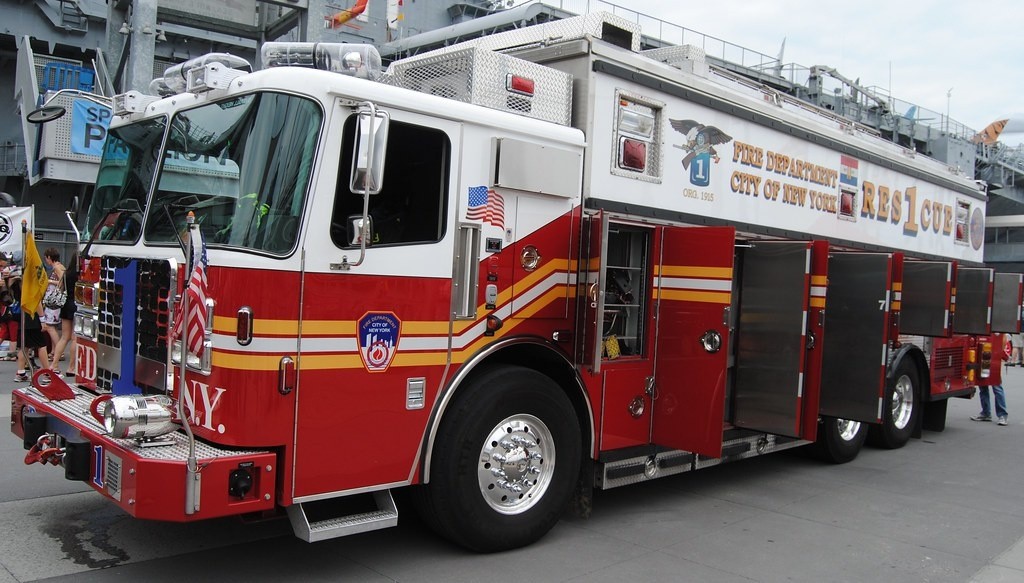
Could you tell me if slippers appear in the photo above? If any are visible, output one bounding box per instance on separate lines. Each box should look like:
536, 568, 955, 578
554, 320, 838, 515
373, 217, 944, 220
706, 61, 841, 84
66, 372, 75, 377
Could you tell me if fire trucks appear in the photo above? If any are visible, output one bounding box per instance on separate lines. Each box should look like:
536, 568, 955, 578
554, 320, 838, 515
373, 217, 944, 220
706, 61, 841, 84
7, 0, 1024, 556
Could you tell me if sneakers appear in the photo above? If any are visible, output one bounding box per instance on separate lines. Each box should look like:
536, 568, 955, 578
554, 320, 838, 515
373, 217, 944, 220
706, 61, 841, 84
14, 373, 31, 382
42, 372, 64, 382
997, 415, 1008, 425
970, 413, 992, 421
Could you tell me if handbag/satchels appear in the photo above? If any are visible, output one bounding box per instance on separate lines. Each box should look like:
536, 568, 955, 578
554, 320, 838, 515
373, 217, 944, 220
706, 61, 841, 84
45, 274, 68, 309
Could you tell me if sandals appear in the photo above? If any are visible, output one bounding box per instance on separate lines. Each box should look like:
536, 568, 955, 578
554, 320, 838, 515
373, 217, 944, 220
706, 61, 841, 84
0, 356, 17, 361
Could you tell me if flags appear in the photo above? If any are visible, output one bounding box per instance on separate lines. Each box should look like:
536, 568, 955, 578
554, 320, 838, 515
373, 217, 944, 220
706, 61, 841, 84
21, 230, 49, 321
171, 224, 208, 358
0, 206, 32, 264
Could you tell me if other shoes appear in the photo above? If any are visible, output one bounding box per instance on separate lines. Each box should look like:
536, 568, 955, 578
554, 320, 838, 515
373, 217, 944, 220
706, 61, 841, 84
49, 353, 64, 360
26, 364, 41, 372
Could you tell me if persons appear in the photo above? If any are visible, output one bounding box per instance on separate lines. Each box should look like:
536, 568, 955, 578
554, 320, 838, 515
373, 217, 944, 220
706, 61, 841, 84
970, 333, 1013, 426
0, 247, 81, 385
